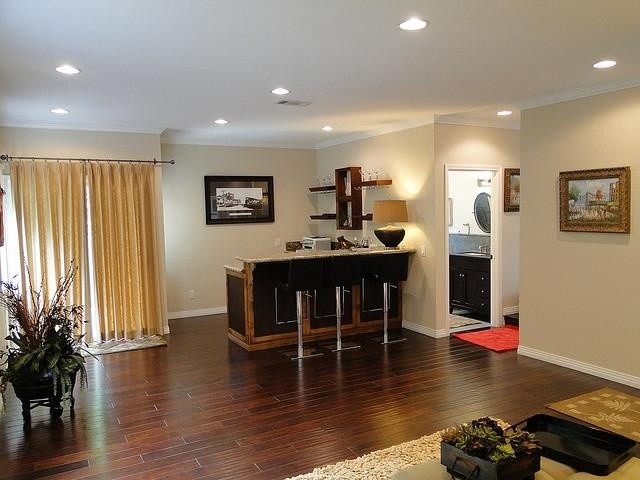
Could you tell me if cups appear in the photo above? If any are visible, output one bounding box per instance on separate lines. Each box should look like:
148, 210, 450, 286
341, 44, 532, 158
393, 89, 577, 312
374, 168, 384, 179
365, 168, 374, 181
358, 169, 367, 182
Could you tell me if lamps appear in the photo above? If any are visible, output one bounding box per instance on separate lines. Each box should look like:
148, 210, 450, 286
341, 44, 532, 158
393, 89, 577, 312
372, 199, 409, 249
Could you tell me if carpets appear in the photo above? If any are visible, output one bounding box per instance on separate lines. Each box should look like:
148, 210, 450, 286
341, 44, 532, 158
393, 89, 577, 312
283, 417, 525, 480
543, 386, 640, 442
451, 323, 519, 353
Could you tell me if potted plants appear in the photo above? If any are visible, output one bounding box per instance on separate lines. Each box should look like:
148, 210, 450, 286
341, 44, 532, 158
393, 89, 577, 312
438, 415, 543, 479
0, 256, 99, 423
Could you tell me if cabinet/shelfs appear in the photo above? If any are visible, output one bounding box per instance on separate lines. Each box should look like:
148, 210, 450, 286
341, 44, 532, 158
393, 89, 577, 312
449, 250, 491, 318
309, 166, 393, 230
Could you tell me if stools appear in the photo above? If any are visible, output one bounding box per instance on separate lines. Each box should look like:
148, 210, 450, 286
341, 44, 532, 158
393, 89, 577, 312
273, 276, 325, 359
368, 271, 407, 345
321, 275, 364, 351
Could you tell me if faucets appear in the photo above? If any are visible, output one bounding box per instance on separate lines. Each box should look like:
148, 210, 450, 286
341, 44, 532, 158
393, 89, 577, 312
479, 245, 488, 254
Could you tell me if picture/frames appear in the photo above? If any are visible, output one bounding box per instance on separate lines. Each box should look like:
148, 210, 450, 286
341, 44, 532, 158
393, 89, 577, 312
503, 167, 520, 210
558, 166, 631, 234
203, 175, 275, 225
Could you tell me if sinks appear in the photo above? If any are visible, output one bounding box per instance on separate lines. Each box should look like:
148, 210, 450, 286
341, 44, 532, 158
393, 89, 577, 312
457, 251, 488, 258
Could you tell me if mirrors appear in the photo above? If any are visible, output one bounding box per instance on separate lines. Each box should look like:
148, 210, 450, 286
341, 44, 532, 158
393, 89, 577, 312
475, 192, 490, 233
